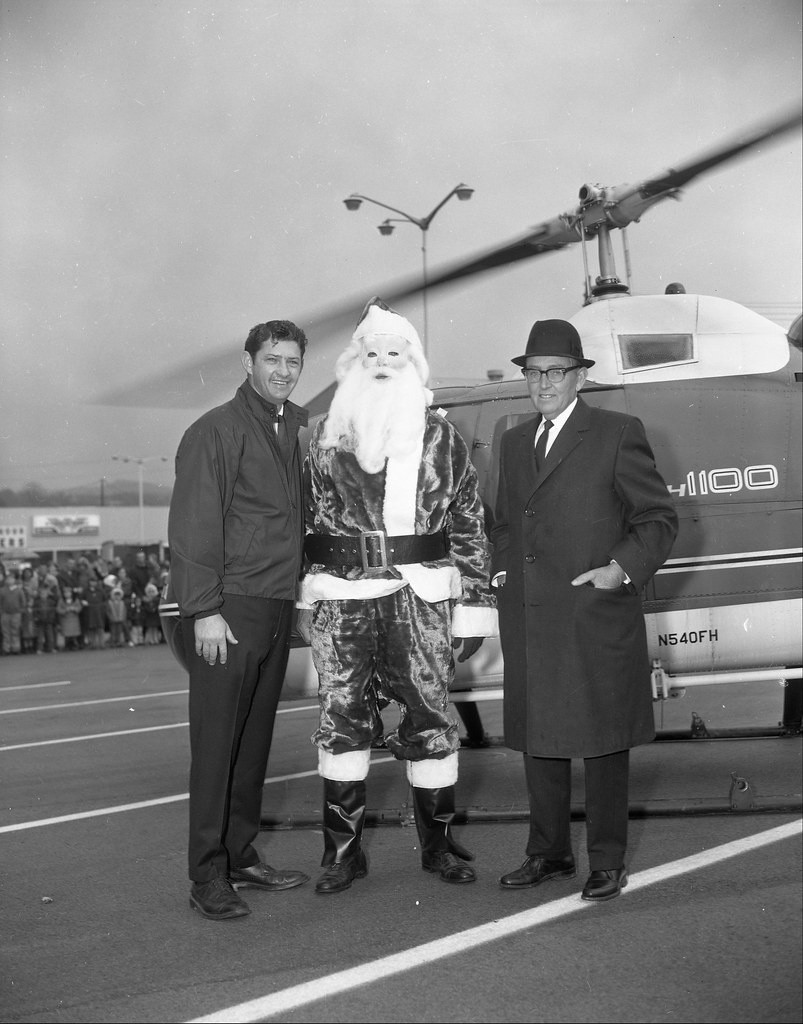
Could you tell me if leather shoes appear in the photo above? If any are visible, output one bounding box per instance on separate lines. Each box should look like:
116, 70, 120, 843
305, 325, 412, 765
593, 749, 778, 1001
500, 849, 575, 889
226, 862, 311, 891
188, 878, 251, 920
582, 863, 627, 900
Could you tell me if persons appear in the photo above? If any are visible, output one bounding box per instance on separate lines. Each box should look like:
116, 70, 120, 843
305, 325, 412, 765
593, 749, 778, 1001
492, 320, 677, 899
168, 320, 308, 922
302, 297, 501, 894
0, 550, 169, 654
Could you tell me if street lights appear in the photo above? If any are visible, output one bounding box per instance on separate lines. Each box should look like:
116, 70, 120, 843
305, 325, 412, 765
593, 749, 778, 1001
338, 177, 471, 374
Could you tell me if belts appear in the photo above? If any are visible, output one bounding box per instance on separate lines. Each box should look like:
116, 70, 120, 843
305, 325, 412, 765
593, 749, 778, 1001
305, 527, 452, 571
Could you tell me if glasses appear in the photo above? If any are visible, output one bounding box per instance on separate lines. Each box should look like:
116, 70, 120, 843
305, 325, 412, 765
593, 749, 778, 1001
521, 363, 581, 382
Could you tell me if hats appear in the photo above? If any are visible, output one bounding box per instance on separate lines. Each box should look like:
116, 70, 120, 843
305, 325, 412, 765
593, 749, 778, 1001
510, 318, 596, 368
352, 294, 424, 345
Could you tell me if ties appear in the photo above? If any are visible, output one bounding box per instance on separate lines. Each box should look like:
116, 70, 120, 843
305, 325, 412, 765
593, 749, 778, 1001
535, 419, 553, 472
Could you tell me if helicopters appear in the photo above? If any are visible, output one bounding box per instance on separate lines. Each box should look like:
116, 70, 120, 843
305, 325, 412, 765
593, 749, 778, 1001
98, 88, 799, 826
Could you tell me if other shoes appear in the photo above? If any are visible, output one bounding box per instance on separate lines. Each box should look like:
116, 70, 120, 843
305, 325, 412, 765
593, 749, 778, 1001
0, 632, 166, 655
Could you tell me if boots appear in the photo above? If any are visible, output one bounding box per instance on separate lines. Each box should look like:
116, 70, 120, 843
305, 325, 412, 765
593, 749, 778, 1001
406, 752, 476, 883
314, 747, 371, 893
